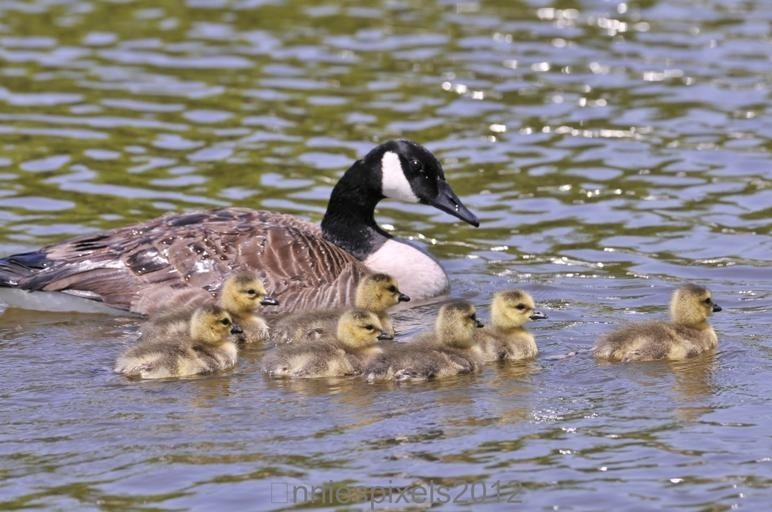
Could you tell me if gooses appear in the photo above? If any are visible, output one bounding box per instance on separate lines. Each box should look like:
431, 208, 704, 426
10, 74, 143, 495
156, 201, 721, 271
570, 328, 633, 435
0, 138, 548, 382
590, 284, 722, 364
